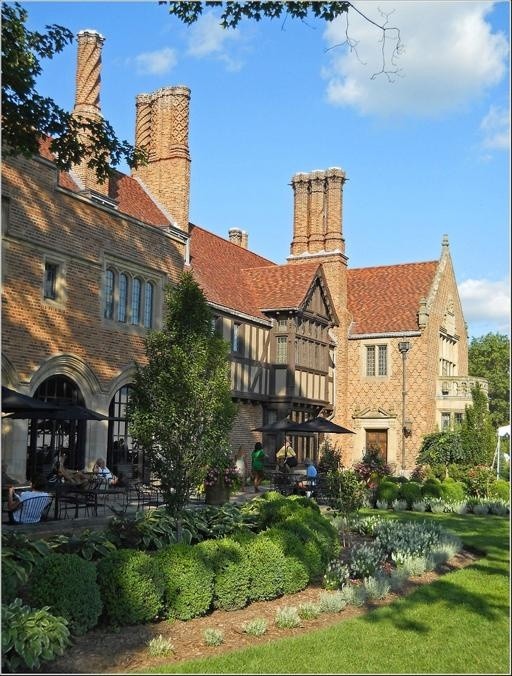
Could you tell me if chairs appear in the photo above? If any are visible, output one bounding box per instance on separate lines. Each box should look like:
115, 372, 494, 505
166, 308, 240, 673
134, 482, 164, 512
263, 470, 327, 505
57, 476, 107, 520
3, 495, 54, 526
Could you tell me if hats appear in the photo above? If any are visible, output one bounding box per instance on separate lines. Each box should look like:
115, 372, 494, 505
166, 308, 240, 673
303, 456, 315, 468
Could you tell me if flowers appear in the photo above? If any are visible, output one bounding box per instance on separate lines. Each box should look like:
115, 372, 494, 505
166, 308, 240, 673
202, 456, 238, 487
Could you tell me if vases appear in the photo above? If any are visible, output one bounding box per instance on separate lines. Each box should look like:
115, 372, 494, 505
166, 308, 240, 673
204, 485, 231, 504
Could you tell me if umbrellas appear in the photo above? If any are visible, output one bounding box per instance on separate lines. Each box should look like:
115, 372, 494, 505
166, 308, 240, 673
1, 386, 66, 413
279, 417, 357, 463
250, 418, 299, 473
1, 403, 118, 516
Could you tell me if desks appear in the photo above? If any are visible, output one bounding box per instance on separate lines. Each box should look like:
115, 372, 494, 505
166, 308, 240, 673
70, 488, 123, 517
83, 472, 108, 489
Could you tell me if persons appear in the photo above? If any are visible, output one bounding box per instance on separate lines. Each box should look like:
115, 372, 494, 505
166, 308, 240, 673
251, 442, 270, 492
8, 471, 49, 525
275, 440, 295, 473
92, 458, 119, 485
296, 456, 318, 489
